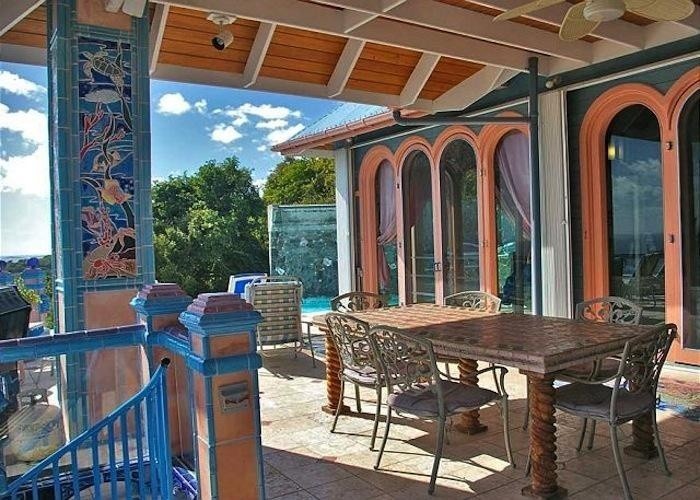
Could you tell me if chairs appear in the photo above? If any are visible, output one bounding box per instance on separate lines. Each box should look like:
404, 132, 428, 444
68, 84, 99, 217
330, 291, 502, 398
22, 322, 55, 387
519, 297, 642, 450
243, 275, 319, 373
226, 272, 268, 299
621, 252, 664, 310
326, 312, 450, 450
523, 321, 678, 497
366, 327, 515, 496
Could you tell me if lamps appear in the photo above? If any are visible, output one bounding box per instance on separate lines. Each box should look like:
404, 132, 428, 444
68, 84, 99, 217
205, 10, 241, 50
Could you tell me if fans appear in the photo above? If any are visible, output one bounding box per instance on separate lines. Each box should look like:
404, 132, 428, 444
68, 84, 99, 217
491, 0, 698, 42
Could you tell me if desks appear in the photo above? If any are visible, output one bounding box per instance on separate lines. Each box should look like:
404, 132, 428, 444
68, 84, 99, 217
312, 304, 663, 498
